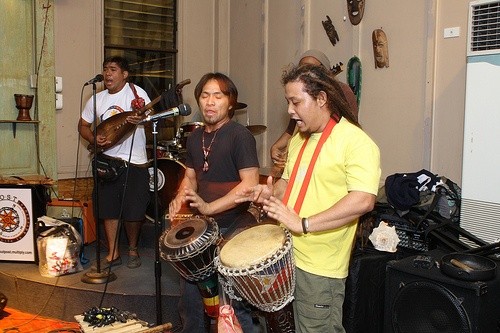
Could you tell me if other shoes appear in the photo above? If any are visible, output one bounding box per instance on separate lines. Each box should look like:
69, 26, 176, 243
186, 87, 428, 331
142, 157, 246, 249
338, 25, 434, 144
127, 247, 142, 268
90, 255, 122, 271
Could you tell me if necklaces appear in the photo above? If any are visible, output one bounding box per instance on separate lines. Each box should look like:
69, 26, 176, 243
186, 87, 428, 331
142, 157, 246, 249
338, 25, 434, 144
202, 118, 230, 172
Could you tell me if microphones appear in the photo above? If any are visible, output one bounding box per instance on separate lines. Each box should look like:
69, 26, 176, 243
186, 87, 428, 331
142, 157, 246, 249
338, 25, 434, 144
84, 74, 104, 86
139, 103, 192, 123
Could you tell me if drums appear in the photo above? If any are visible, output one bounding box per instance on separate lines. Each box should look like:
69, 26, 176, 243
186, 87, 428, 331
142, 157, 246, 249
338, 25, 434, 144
145, 144, 167, 160
158, 214, 223, 333
156, 139, 180, 152
144, 156, 186, 224
176, 121, 204, 156
14, 94, 34, 121
217, 224, 296, 333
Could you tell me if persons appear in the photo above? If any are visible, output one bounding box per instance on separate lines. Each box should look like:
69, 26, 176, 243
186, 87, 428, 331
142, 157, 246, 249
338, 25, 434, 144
169, 72, 260, 333
270, 49, 358, 169
235, 63, 381, 333
78, 55, 156, 271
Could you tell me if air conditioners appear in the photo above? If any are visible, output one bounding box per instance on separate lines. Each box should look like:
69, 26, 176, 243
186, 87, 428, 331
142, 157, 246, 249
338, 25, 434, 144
459, 0, 500, 258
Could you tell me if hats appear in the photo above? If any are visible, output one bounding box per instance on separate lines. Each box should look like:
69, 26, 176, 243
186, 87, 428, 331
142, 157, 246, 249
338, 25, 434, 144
297, 49, 331, 71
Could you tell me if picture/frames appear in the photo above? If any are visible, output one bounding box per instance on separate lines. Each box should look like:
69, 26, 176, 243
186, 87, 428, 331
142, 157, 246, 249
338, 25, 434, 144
0, 184, 39, 265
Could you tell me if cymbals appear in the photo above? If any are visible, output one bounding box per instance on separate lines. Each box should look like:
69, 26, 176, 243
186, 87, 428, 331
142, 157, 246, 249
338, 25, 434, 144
234, 102, 248, 110
245, 125, 267, 136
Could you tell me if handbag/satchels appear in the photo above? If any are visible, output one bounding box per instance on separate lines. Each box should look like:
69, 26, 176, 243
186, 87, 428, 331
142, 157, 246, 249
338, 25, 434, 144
92, 151, 128, 182
36, 224, 84, 278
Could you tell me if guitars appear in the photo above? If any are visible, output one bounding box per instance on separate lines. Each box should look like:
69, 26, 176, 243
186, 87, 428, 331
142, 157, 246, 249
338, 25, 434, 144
271, 62, 344, 175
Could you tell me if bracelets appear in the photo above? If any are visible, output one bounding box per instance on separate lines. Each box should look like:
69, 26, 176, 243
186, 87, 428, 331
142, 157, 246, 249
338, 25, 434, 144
302, 217, 310, 234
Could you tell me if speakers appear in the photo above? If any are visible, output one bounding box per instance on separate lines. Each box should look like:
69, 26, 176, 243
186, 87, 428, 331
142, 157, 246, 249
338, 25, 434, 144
46, 198, 98, 245
383, 247, 500, 333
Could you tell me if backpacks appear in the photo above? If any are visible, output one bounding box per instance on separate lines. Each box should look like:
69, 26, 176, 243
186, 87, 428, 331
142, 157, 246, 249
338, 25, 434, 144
384, 169, 462, 225
